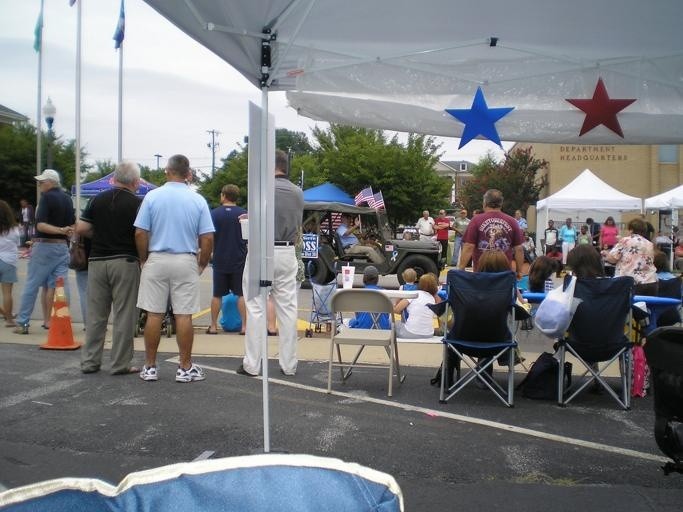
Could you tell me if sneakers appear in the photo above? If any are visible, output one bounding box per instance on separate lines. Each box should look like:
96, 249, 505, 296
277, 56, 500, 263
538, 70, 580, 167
140, 365, 158, 381
12, 325, 29, 335
175, 364, 205, 382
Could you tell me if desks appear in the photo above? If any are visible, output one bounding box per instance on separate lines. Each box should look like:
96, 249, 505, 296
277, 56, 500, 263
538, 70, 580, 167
438, 290, 681, 340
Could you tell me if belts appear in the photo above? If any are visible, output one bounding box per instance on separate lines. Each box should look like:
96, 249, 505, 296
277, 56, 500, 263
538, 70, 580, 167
33, 238, 67, 244
274, 240, 294, 247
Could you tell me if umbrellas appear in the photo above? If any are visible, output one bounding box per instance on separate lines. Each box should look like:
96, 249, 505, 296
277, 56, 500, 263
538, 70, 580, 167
71, 170, 159, 200
303, 181, 380, 214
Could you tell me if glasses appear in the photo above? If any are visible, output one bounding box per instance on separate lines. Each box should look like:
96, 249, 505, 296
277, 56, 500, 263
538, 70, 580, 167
436, 211, 480, 216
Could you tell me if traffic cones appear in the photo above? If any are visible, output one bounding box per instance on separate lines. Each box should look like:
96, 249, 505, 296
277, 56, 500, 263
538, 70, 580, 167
36, 276, 82, 352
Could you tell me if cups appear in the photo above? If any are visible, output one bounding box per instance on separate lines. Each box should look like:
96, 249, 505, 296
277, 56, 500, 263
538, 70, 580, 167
342, 266, 355, 290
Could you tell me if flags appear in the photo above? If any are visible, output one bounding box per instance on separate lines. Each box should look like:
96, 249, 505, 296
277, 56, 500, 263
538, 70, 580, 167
33, 0, 43, 53
113, 0, 125, 49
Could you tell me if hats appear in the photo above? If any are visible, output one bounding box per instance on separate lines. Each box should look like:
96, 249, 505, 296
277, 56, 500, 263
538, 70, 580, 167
33, 168, 61, 182
363, 265, 379, 280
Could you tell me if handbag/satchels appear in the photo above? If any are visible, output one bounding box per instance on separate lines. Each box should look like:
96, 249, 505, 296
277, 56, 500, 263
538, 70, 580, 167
521, 351, 573, 399
625, 347, 650, 397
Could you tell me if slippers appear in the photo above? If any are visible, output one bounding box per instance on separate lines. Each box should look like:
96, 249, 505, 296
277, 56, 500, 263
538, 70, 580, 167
207, 327, 278, 335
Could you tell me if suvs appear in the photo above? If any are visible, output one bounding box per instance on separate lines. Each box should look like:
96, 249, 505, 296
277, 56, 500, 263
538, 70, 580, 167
301, 201, 445, 289
393, 228, 419, 242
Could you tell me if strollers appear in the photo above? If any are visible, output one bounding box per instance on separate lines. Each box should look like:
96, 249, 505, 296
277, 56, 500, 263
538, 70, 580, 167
303, 261, 345, 338
135, 290, 175, 339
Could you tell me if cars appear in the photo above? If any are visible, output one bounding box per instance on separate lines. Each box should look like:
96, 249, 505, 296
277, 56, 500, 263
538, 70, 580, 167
446, 215, 457, 229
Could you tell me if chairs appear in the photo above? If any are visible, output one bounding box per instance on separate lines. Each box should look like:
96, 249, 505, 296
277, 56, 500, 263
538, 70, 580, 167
557, 274, 635, 410
327, 289, 444, 396
439, 270, 517, 408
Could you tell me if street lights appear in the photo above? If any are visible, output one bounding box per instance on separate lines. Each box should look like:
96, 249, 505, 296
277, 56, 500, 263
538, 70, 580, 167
42, 98, 56, 170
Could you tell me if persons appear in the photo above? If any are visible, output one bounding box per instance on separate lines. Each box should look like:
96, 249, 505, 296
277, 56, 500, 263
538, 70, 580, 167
267, 224, 305, 335
218, 289, 242, 332
133, 155, 216, 383
236, 149, 303, 376
0, 169, 108, 333
76, 163, 142, 375
206, 184, 248, 335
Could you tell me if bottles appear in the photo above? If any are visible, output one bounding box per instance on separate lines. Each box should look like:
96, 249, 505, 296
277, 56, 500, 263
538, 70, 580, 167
521, 299, 534, 330
563, 361, 573, 391
